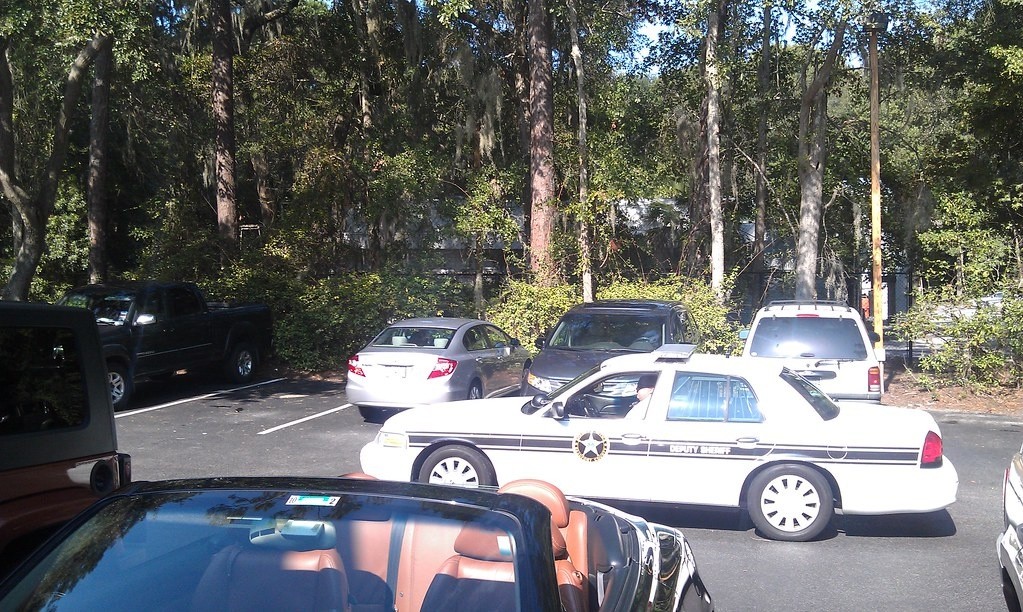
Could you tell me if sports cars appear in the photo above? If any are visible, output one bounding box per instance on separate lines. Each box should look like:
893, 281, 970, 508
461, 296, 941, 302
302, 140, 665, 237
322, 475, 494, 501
0, 472, 717, 612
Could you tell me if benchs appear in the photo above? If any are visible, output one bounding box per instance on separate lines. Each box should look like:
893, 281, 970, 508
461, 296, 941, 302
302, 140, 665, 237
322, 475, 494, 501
389, 479, 590, 612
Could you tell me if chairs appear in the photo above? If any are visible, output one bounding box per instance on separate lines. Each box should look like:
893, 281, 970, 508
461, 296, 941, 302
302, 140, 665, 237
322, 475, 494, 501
188, 521, 352, 612
574, 322, 612, 346
392, 336, 407, 345
434, 338, 448, 347
419, 521, 587, 612
135, 299, 160, 325
335, 472, 401, 612
639, 325, 659, 346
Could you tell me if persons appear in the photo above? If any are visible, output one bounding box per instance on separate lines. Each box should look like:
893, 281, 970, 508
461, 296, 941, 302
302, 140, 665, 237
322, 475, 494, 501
624, 375, 658, 421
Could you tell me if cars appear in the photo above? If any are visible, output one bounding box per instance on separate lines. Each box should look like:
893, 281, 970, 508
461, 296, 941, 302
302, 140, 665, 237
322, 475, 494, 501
737, 298, 888, 408
345, 314, 535, 418
360, 342, 959, 543
520, 297, 703, 404
995, 438, 1023, 612
0, 297, 131, 562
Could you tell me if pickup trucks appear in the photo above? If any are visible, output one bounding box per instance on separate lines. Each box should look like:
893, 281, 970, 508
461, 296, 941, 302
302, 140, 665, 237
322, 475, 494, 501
50, 279, 277, 413
